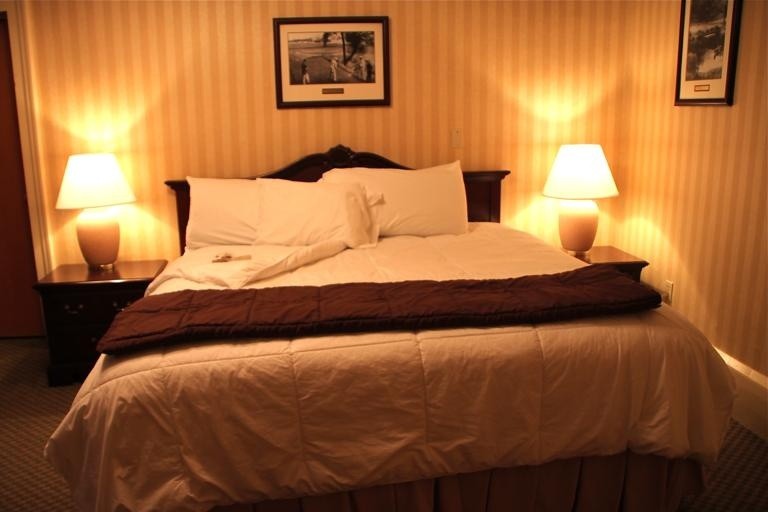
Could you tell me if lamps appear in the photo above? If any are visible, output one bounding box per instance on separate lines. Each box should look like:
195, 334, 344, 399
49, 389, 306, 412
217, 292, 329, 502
537, 143, 620, 261
56, 150, 132, 273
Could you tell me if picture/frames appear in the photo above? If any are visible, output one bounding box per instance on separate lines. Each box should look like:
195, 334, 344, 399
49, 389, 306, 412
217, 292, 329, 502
673, 0, 745, 107
272, 16, 391, 108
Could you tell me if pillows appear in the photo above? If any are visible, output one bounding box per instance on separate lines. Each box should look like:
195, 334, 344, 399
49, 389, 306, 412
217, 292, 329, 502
325, 158, 471, 239
252, 170, 373, 246
184, 177, 260, 248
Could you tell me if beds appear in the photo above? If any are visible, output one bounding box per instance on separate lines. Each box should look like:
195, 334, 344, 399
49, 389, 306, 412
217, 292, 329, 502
42, 136, 741, 511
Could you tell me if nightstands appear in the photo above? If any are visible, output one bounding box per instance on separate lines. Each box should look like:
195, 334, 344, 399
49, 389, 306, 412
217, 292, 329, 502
568, 241, 654, 291
35, 260, 166, 388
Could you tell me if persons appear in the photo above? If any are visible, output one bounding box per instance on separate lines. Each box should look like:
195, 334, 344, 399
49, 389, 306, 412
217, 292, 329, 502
350, 57, 359, 80
300, 59, 311, 86
363, 60, 374, 81
328, 55, 338, 84
